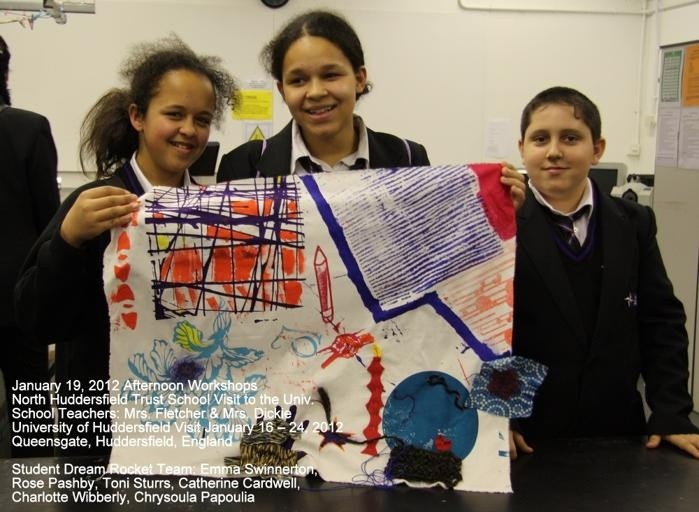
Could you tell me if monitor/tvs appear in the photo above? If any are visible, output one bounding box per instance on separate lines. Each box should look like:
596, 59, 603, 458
587, 162, 628, 199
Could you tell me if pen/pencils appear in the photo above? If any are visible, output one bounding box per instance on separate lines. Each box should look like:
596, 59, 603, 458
314, 245, 334, 323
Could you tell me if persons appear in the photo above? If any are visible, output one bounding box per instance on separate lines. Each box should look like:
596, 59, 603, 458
0, 37, 63, 457
503, 86, 699, 459
15, 31, 239, 460
217, 8, 528, 215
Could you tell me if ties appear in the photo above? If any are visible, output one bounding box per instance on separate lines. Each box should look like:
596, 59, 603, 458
557, 207, 586, 248
